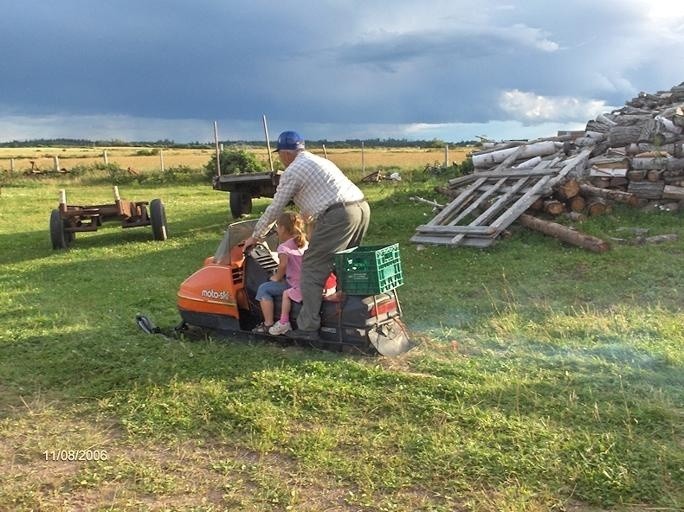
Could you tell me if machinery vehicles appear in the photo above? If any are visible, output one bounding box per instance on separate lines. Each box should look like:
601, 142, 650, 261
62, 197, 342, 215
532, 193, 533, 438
129, 211, 420, 359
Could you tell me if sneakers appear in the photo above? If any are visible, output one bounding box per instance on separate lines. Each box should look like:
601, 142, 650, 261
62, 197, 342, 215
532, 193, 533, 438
250, 322, 276, 334
283, 328, 321, 342
268, 321, 293, 337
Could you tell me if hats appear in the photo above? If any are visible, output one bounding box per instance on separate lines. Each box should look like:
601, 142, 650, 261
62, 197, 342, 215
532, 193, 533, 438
269, 130, 306, 154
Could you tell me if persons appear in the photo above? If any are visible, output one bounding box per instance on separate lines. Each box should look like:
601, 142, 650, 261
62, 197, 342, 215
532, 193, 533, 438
266, 210, 310, 337
305, 214, 337, 298
240, 129, 371, 340
249, 269, 292, 335
